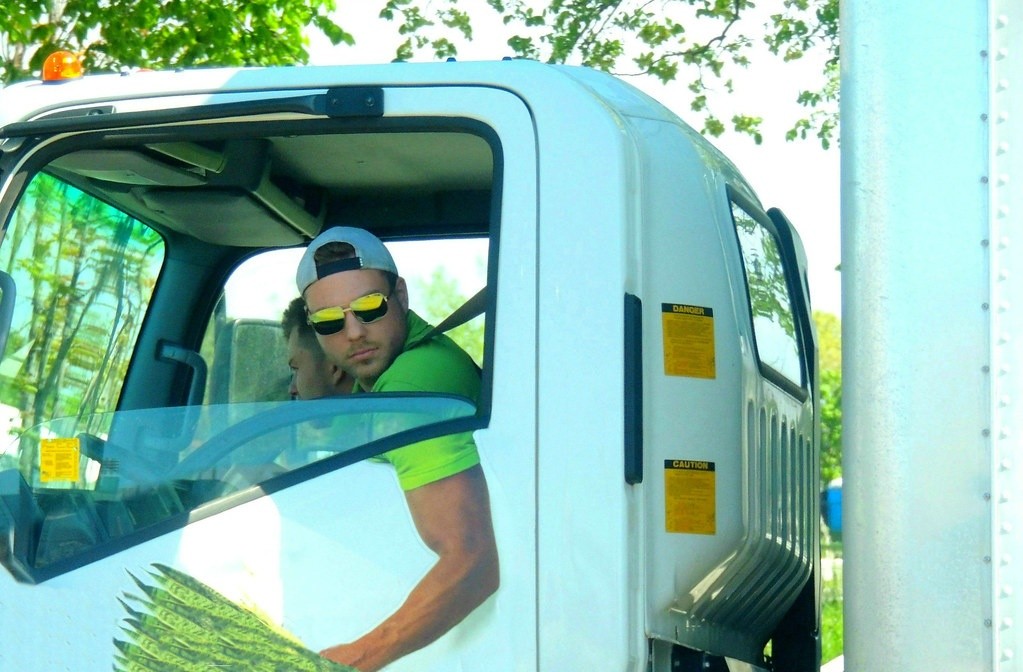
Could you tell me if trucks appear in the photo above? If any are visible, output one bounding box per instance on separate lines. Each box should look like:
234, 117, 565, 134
0, 0, 1023, 670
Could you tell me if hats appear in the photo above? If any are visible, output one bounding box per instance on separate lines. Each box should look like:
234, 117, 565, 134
297, 226, 398, 299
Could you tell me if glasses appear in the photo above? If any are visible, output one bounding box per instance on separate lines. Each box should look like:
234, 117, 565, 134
307, 286, 395, 336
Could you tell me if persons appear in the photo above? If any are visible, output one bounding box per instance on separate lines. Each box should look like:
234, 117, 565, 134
274, 290, 373, 456
296, 217, 491, 447
313, 428, 504, 670
